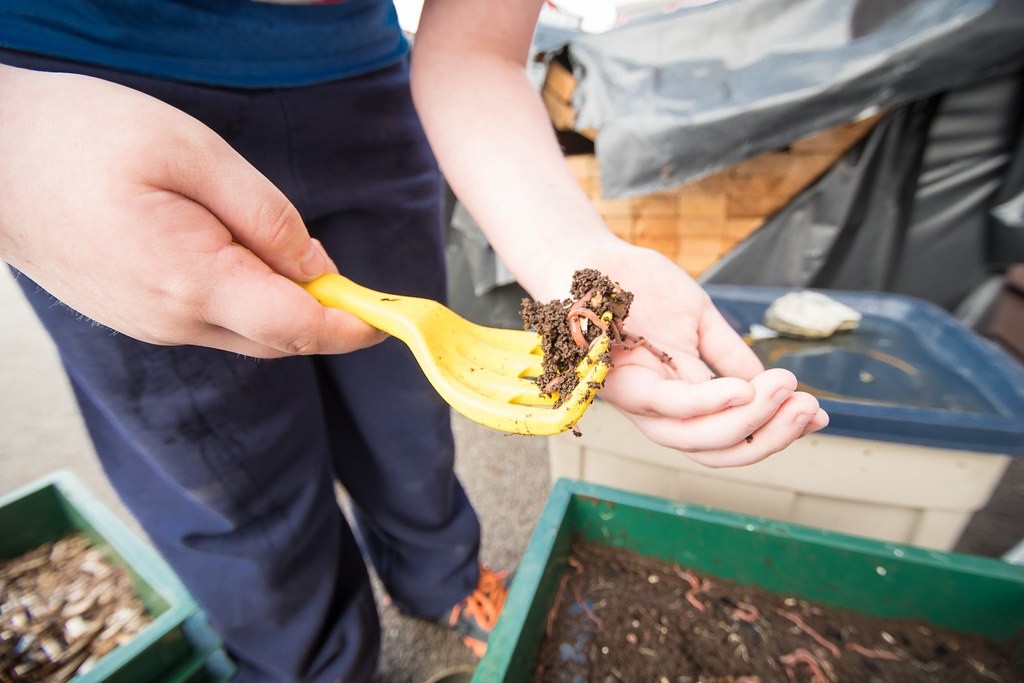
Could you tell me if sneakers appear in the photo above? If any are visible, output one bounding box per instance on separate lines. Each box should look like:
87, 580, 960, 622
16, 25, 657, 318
433, 562, 510, 657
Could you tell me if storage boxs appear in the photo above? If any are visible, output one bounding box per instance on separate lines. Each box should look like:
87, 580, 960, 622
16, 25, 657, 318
539, 282, 1023, 551
0, 471, 232, 683
470, 475, 1024, 683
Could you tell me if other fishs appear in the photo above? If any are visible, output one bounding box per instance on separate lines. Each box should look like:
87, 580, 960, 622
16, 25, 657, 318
0, 529, 155, 683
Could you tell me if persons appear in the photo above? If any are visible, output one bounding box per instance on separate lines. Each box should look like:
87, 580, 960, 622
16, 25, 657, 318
0, 0, 832, 683
